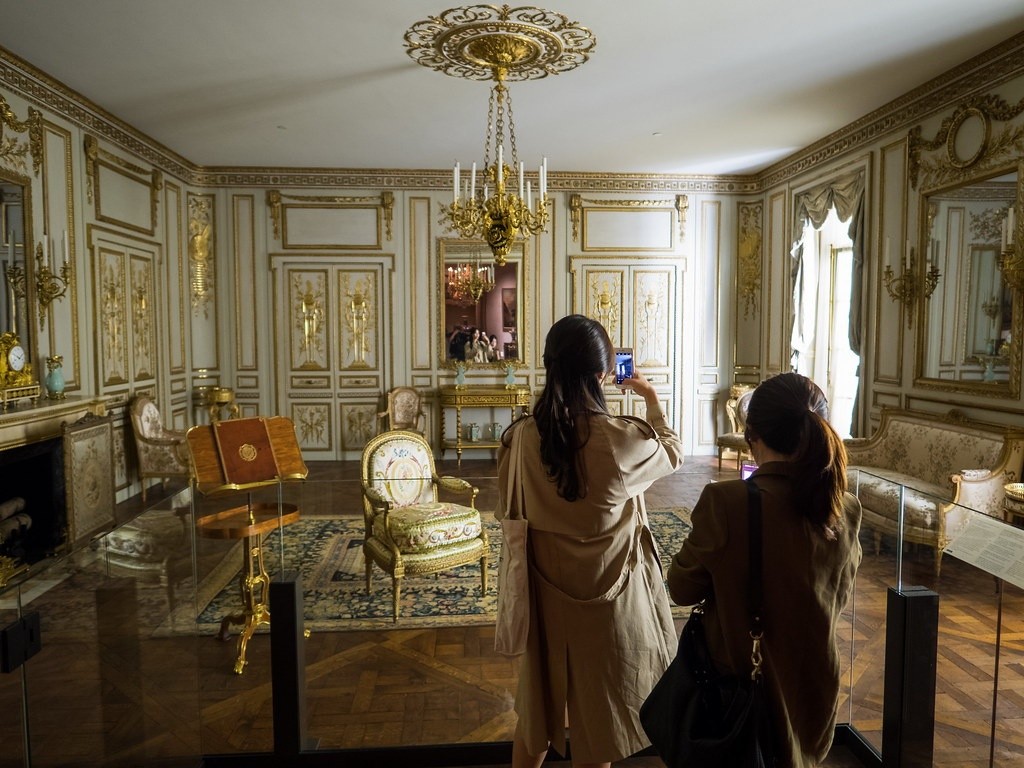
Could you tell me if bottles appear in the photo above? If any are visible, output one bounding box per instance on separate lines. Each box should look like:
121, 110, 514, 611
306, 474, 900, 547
44, 356, 65, 400
488, 423, 502, 441
467, 423, 480, 441
984, 364, 995, 383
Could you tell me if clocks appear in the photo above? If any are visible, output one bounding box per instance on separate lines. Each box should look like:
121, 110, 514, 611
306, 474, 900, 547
0, 331, 34, 385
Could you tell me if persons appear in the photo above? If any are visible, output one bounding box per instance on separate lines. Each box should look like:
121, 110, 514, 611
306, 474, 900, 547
664, 372, 863, 768
446, 324, 502, 364
494, 314, 685, 768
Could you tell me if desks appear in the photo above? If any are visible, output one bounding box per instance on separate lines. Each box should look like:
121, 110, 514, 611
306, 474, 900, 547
439, 382, 531, 460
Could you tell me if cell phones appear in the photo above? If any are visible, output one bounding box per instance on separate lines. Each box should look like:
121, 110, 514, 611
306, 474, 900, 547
740, 465, 759, 480
614, 347, 635, 389
481, 332, 486, 336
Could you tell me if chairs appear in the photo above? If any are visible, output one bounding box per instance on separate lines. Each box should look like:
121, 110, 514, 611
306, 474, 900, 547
716, 383, 757, 472
359, 431, 489, 624
130, 391, 206, 494
376, 387, 432, 443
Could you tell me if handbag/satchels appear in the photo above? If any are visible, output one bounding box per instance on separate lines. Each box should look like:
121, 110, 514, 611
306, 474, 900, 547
640, 479, 770, 768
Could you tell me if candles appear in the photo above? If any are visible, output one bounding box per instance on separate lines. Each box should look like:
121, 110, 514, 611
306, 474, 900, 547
936, 240, 940, 269
931, 239, 936, 267
1007, 206, 1014, 245
63, 230, 69, 263
43, 235, 48, 266
1001, 220, 1007, 253
886, 236, 891, 268
906, 239, 911, 271
8, 230, 14, 268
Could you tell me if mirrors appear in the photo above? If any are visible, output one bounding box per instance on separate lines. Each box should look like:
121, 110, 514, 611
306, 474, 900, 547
0, 167, 39, 386
912, 119, 1024, 401
435, 236, 530, 370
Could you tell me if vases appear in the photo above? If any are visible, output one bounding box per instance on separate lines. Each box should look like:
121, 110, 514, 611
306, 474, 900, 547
44, 354, 67, 401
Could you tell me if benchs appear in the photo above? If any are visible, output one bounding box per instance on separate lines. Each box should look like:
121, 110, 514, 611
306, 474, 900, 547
835, 405, 1024, 577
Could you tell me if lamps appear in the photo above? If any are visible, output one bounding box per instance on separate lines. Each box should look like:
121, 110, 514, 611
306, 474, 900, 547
403, 4, 597, 264
447, 247, 495, 304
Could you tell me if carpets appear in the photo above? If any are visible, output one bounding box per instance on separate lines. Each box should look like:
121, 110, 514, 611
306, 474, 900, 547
147, 505, 705, 636
